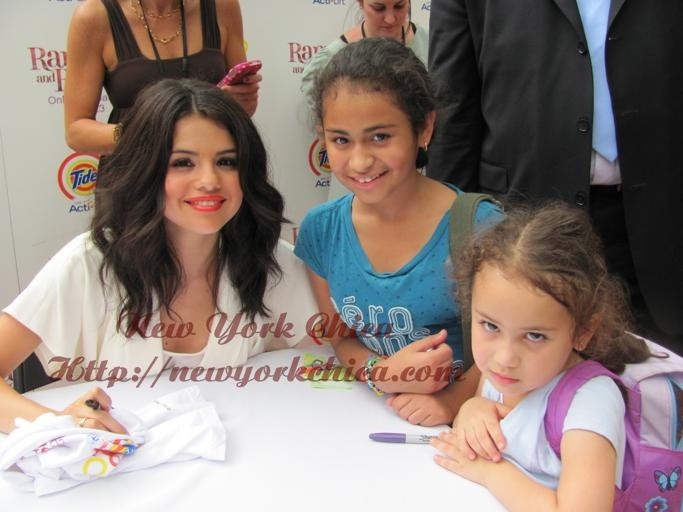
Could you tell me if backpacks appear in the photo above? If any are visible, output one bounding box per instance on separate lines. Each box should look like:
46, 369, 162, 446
544, 330, 683, 512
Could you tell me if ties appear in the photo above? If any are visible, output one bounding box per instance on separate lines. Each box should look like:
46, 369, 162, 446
578, 1, 618, 164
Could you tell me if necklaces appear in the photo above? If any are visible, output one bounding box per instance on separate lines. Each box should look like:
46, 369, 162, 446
133, 1, 184, 43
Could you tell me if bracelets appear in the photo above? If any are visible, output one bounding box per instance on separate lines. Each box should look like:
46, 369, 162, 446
360, 350, 391, 398
112, 124, 121, 146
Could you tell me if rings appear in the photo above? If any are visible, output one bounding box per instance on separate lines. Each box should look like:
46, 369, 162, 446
78, 416, 89, 427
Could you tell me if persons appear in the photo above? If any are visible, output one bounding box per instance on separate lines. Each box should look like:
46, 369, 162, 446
428, 198, 682, 511
423, 2, 683, 353
299, 0, 431, 142
0, 77, 321, 439
63, 0, 260, 155
293, 36, 509, 427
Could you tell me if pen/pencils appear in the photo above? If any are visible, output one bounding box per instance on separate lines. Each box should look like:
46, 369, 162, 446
85, 399, 103, 410
369, 433, 436, 444
